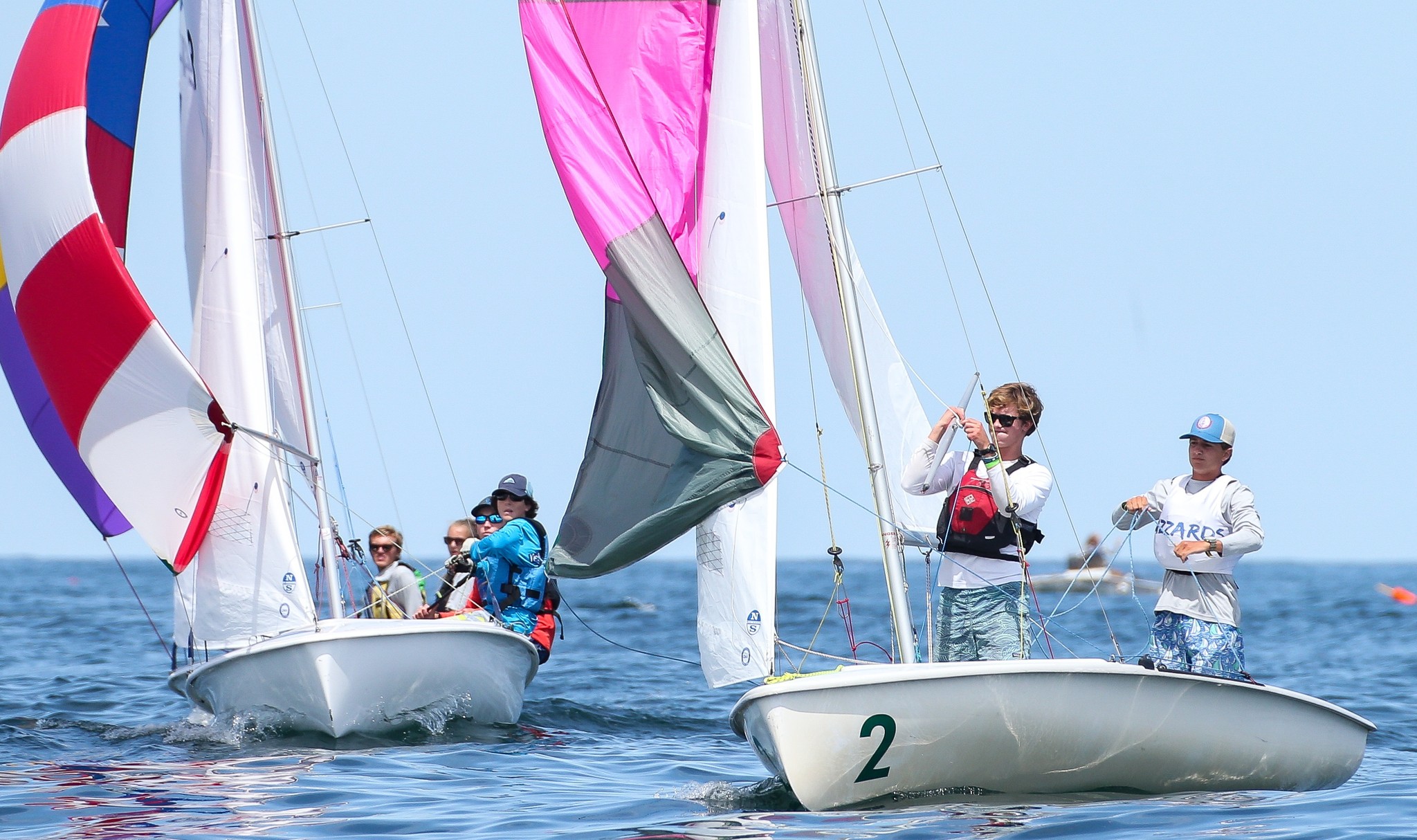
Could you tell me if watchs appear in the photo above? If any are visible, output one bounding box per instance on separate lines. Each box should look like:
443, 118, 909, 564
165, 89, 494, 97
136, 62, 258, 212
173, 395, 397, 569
1203, 536, 1217, 558
979, 443, 996, 456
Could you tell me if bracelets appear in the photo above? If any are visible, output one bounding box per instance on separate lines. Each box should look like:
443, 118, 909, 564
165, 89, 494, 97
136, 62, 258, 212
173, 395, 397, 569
981, 452, 1000, 469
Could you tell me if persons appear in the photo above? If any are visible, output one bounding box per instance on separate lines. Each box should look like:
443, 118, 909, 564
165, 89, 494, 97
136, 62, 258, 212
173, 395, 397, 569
902, 384, 1052, 662
445, 475, 548, 637
415, 496, 556, 665
1112, 413, 1264, 681
438, 518, 478, 606
365, 525, 423, 619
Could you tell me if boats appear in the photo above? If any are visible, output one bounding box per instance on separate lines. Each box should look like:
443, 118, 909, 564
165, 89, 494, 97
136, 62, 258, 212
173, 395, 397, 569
1022, 565, 1163, 603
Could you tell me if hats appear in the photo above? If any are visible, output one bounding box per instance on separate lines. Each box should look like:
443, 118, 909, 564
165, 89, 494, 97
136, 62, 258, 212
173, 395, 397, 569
492, 474, 534, 499
471, 496, 498, 518
1179, 413, 1236, 447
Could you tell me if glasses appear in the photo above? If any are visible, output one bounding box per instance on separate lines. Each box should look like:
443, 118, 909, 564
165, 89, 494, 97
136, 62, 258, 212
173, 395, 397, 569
496, 492, 525, 502
984, 411, 1019, 428
444, 536, 466, 546
475, 514, 503, 524
370, 542, 394, 552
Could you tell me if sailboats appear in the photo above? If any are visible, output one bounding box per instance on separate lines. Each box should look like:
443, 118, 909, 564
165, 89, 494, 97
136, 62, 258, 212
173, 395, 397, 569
0, 0, 542, 746
501, 0, 1381, 818
